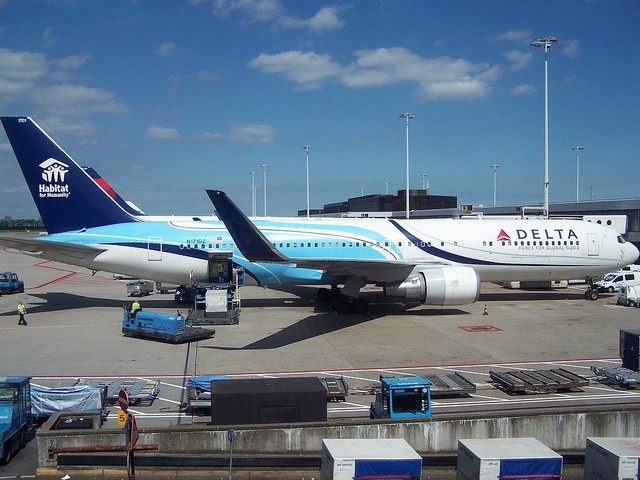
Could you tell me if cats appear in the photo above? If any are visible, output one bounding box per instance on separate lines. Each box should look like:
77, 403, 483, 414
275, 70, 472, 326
0, 115, 640, 318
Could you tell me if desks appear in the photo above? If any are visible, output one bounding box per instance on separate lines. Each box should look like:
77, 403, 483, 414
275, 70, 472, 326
259, 164, 271, 217
360, 186, 366, 195
249, 170, 257, 216
529, 36, 561, 219
570, 146, 586, 200
489, 163, 501, 208
384, 180, 389, 195
398, 111, 417, 219
419, 173, 428, 189
302, 144, 316, 217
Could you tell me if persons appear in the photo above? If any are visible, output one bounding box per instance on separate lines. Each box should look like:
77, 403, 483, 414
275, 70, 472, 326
130, 299, 142, 316
176, 310, 182, 316
216, 260, 225, 283
17, 300, 27, 325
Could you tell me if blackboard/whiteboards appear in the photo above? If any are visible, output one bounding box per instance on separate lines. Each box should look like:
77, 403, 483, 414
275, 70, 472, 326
0, 271, 25, 295
369, 372, 432, 419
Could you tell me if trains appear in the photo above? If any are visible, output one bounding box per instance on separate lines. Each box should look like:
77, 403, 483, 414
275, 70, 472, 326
118, 388, 130, 411
